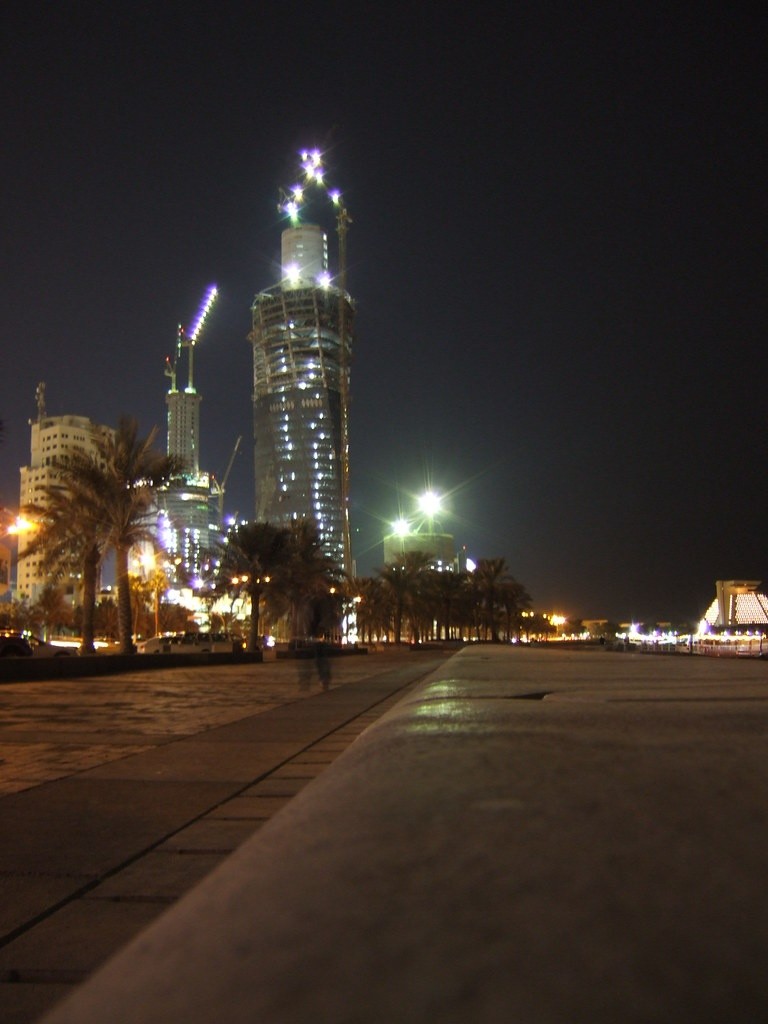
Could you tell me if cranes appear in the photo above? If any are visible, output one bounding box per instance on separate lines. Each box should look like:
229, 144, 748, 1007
211, 435, 242, 532
180, 287, 219, 393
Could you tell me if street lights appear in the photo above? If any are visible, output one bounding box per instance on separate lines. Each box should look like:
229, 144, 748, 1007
9, 517, 51, 644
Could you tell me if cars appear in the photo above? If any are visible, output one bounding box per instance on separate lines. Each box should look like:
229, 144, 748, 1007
0, 630, 77, 658
137, 637, 210, 654
